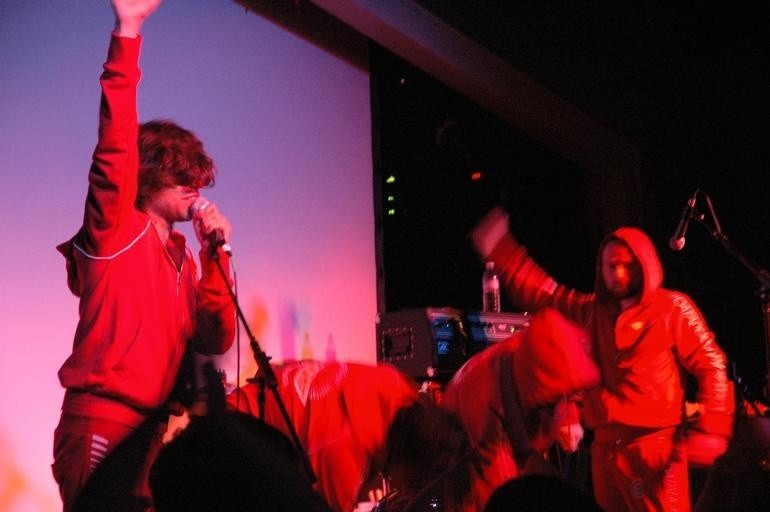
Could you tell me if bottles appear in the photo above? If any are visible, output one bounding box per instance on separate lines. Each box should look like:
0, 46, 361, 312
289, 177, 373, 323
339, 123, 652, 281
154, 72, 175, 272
482, 261, 501, 314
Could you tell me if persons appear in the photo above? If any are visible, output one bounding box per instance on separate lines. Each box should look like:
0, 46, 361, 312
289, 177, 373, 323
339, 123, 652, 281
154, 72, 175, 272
146, 208, 770, 512
53, 2, 236, 512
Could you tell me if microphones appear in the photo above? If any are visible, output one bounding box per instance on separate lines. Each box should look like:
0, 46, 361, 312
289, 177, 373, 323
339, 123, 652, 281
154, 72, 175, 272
668, 197, 708, 251
188, 196, 233, 259
